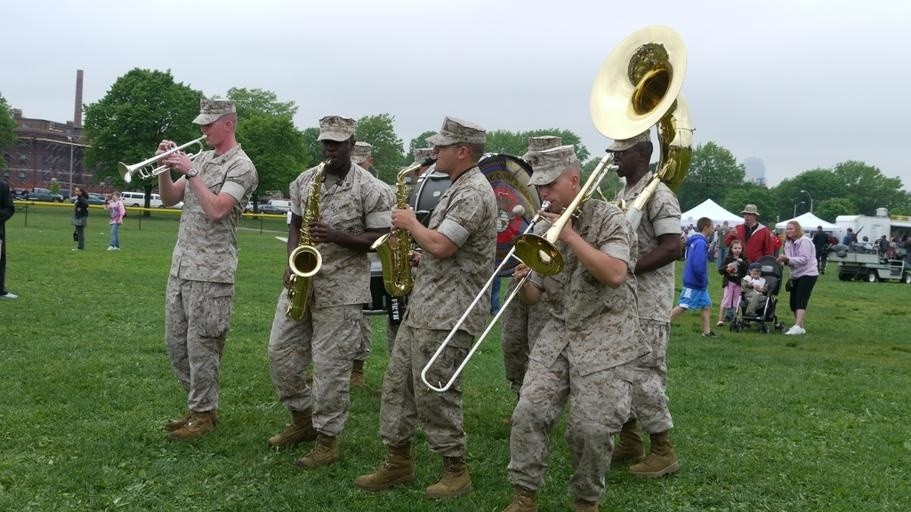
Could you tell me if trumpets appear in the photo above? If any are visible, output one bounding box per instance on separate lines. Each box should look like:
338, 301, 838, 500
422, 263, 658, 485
118, 134, 207, 182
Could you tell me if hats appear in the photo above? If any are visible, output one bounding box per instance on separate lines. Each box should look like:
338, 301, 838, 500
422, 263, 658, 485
749, 262, 761, 269
191, 97, 237, 126
425, 115, 487, 147
740, 205, 761, 216
316, 114, 356, 143
522, 135, 577, 186
416, 147, 436, 162
350, 141, 371, 164
606, 129, 650, 152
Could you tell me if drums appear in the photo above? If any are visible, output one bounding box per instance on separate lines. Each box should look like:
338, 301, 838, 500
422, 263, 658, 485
410, 154, 543, 278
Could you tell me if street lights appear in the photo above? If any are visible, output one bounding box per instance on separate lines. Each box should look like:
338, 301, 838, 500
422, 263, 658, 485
794, 201, 806, 218
801, 189, 814, 213
68, 136, 74, 203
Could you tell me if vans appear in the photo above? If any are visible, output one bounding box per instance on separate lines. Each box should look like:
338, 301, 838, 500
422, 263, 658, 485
120, 192, 170, 209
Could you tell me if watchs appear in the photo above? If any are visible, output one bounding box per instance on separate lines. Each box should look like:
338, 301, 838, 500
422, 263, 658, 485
185, 168, 199, 179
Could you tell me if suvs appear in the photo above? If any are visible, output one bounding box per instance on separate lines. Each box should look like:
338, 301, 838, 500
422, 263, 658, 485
838, 245, 911, 284
13, 187, 71, 202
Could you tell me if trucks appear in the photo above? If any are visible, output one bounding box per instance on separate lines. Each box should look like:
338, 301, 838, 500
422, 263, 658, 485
267, 200, 292, 214
247, 201, 261, 212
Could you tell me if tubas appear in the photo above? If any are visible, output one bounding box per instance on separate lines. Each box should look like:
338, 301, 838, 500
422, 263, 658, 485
595, 25, 692, 230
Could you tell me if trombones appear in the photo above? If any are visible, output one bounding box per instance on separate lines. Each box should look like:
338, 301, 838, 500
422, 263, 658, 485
420, 152, 615, 392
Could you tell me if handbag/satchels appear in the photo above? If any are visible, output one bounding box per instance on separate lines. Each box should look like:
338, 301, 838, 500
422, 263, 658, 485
786, 279, 794, 292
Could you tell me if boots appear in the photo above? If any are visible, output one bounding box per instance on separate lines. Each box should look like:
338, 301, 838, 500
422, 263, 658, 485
503, 484, 538, 512
609, 420, 645, 465
573, 495, 598, 512
165, 410, 219, 442
356, 441, 414, 491
268, 405, 318, 448
425, 456, 472, 500
351, 359, 365, 389
294, 430, 339, 471
629, 428, 679, 479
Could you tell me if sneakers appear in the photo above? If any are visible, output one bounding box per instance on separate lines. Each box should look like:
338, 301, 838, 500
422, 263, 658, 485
717, 316, 731, 327
701, 329, 717, 339
784, 325, 806, 336
108, 245, 120, 251
745, 312, 757, 316
3, 292, 17, 298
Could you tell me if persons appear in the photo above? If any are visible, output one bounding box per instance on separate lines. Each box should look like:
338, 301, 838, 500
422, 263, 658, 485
0, 180, 14, 296
155, 96, 259, 438
776, 220, 818, 336
501, 144, 652, 511
724, 204, 774, 266
610, 129, 682, 477
742, 263, 769, 316
106, 192, 125, 250
718, 239, 748, 327
356, 118, 497, 501
670, 204, 910, 275
671, 217, 717, 339
72, 187, 88, 252
328, 134, 562, 425
267, 117, 390, 468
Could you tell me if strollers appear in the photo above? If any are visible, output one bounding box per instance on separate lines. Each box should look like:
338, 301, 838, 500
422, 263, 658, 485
730, 256, 786, 333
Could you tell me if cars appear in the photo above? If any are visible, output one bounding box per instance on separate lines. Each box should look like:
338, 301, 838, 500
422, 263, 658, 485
166, 201, 184, 209
250, 204, 289, 215
71, 193, 110, 205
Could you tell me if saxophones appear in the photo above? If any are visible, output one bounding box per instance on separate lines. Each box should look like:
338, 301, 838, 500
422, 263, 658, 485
371, 160, 437, 298
285, 158, 339, 321
